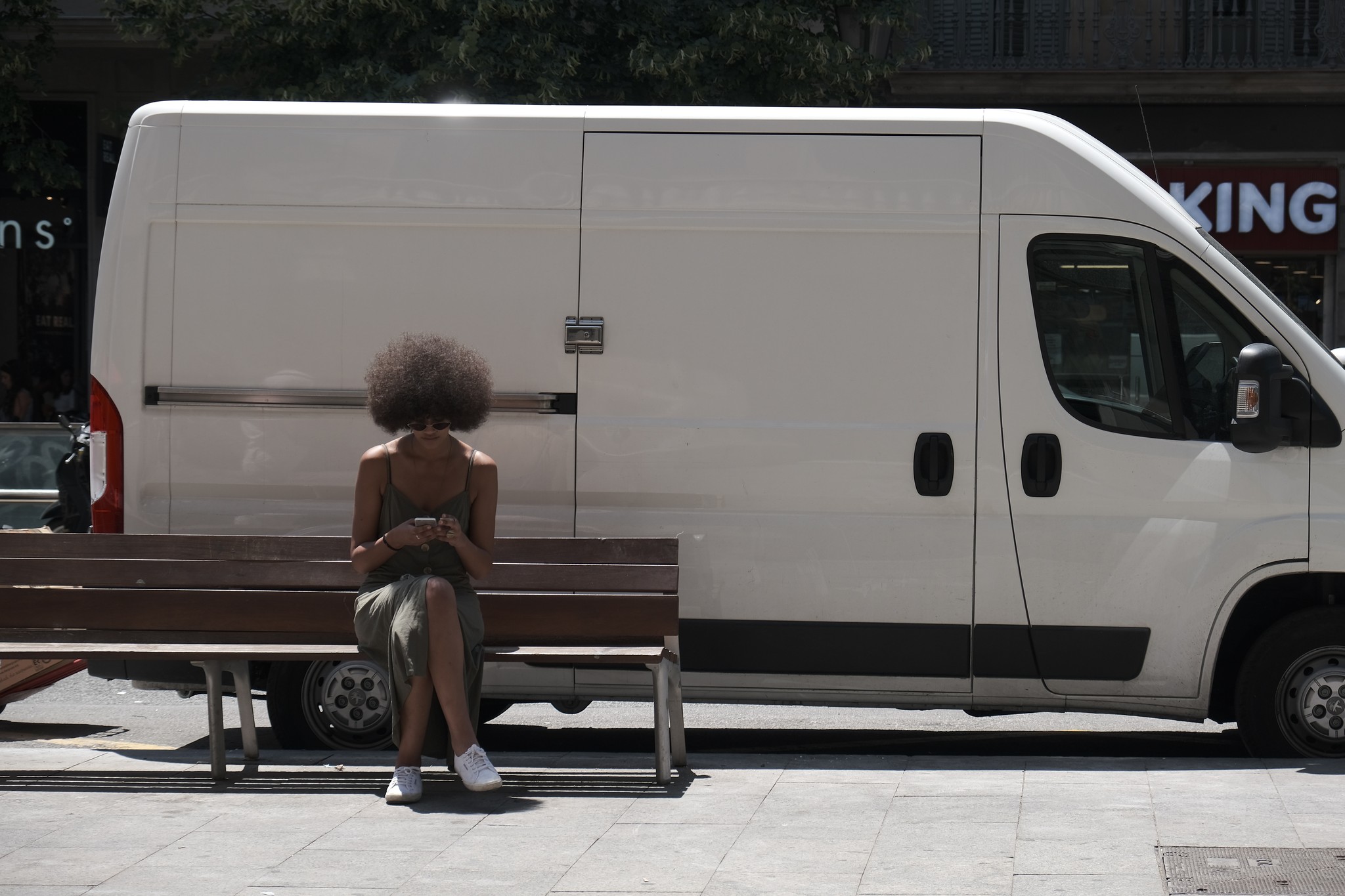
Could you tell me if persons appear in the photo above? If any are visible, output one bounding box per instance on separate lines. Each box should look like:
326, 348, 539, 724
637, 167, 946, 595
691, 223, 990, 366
350, 333, 502, 805
1043, 287, 1113, 425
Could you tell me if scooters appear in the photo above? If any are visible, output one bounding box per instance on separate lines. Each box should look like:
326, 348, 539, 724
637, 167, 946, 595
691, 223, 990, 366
40, 414, 91, 533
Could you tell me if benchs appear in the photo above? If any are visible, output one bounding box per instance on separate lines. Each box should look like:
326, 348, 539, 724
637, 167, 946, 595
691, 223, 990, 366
0, 533, 687, 783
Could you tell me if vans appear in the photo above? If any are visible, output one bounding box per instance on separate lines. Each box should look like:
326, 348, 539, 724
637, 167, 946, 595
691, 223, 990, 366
89, 99, 1345, 760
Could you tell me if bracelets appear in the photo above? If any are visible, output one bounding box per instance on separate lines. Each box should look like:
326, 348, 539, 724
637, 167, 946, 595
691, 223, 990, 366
381, 534, 403, 555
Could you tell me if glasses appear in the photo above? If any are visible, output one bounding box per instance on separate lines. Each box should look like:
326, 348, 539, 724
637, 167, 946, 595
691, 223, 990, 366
407, 421, 452, 431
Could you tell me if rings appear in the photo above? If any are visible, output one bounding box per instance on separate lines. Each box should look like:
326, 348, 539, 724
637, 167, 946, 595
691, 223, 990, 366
446, 528, 454, 539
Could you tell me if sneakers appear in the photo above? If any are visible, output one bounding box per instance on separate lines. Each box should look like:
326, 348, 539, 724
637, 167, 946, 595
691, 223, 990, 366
385, 766, 422, 803
454, 743, 502, 792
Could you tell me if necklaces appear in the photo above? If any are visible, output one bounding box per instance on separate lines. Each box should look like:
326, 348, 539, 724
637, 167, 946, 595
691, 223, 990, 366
409, 433, 452, 493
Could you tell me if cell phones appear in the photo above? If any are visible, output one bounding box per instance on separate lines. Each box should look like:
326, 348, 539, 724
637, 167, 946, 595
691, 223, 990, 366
414, 517, 436, 529
